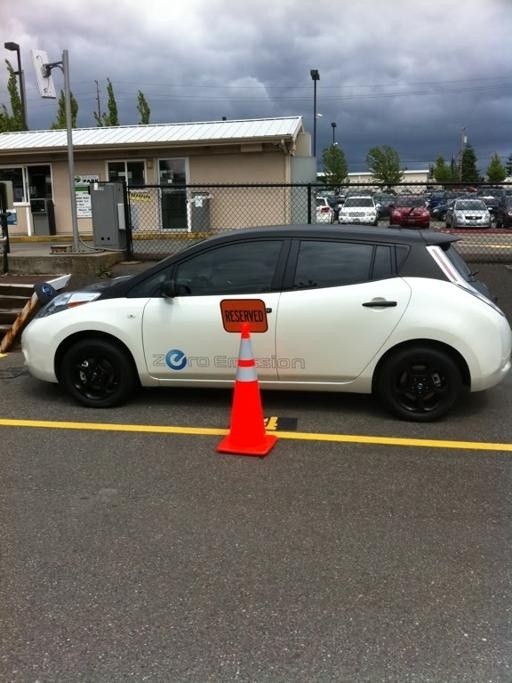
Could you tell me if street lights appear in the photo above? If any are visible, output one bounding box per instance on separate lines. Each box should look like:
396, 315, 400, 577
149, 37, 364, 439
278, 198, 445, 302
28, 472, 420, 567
310, 69, 321, 157
331, 120, 337, 146
4, 41, 28, 131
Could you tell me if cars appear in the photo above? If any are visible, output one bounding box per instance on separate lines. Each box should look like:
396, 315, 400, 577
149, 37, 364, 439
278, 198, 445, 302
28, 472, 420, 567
19, 224, 511, 425
316, 186, 512, 225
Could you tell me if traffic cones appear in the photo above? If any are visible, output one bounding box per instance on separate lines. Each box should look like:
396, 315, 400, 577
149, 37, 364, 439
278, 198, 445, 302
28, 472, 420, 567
214, 318, 278, 457
